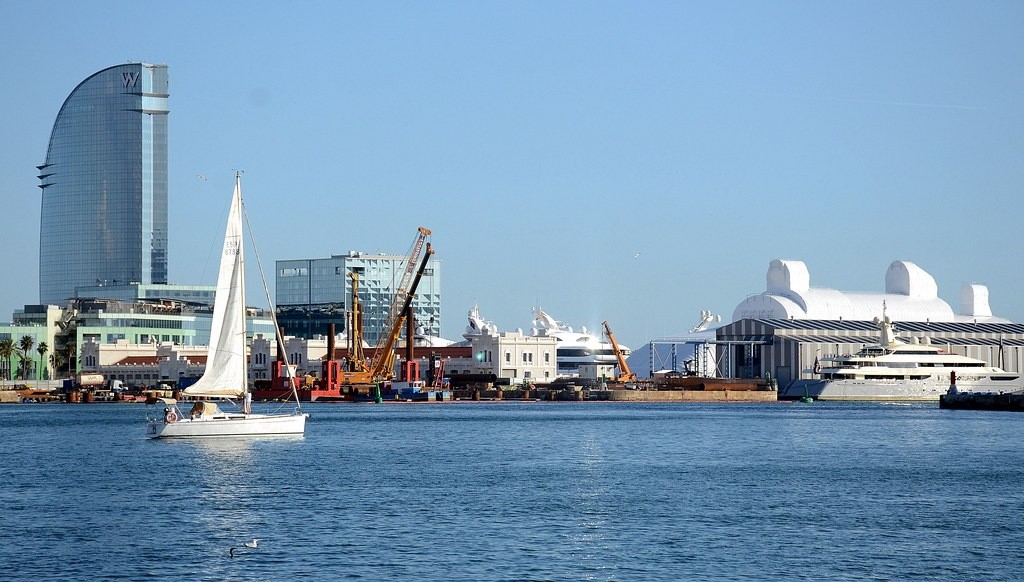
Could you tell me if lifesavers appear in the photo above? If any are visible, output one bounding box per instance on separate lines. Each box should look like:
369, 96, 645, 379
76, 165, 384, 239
166, 412, 177, 423
531, 385, 535, 388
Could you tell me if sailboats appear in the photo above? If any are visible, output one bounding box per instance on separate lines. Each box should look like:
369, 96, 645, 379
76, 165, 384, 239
143, 168, 313, 441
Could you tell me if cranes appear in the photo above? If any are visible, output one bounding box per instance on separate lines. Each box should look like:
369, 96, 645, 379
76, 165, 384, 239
330, 226, 439, 393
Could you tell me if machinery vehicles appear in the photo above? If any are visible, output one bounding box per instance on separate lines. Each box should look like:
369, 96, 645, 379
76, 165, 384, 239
74, 373, 129, 394
601, 320, 639, 386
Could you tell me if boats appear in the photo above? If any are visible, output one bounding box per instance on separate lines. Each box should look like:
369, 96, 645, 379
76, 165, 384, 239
776, 297, 1024, 401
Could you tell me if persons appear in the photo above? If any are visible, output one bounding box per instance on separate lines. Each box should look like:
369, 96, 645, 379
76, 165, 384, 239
246, 390, 252, 414
